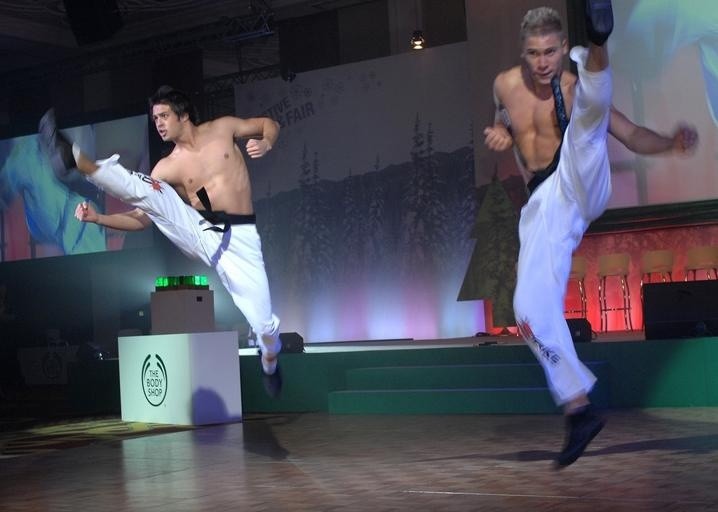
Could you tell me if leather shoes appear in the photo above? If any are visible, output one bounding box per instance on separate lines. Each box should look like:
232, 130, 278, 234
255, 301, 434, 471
550, 403, 607, 471
257, 348, 284, 403
585, 1, 615, 48
36, 106, 79, 180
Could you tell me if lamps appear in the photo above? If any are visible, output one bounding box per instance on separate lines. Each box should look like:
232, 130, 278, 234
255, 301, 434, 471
410, 0, 426, 50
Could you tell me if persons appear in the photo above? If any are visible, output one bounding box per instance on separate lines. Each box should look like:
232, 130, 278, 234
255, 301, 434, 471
0, 124, 110, 258
481, 1, 699, 473
33, 82, 285, 401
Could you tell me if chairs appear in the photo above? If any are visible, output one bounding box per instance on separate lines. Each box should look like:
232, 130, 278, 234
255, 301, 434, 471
562, 244, 718, 335
